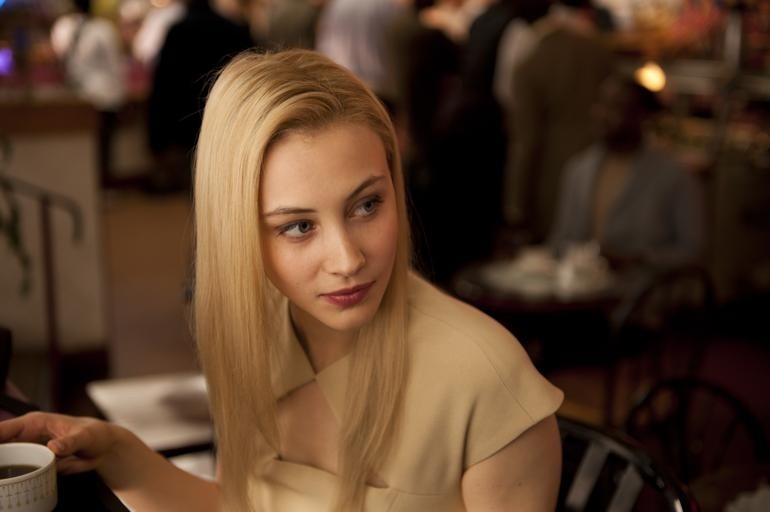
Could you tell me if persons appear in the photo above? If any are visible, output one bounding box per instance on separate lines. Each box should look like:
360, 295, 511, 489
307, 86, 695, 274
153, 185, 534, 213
0, 46, 568, 511
52, 2, 770, 364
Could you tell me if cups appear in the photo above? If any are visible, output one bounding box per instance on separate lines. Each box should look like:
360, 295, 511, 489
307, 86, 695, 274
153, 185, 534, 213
0, 443, 58, 512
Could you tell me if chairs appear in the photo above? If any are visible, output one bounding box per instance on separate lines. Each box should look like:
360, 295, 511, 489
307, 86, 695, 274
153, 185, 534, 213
0, 1, 770, 511
